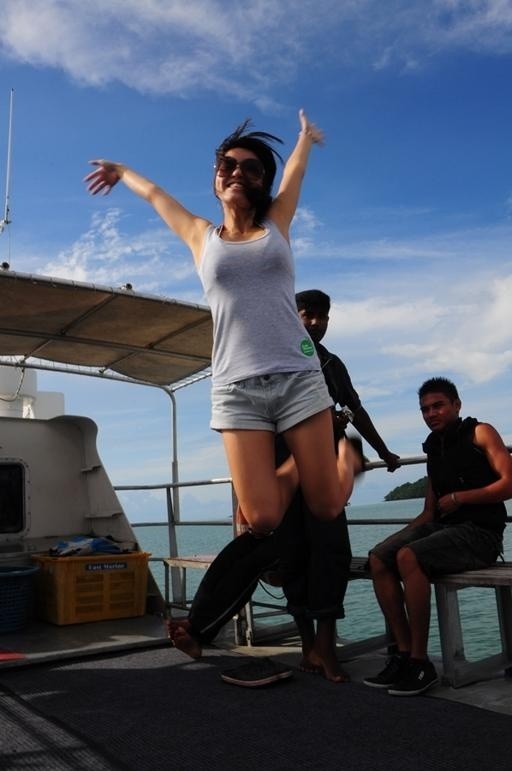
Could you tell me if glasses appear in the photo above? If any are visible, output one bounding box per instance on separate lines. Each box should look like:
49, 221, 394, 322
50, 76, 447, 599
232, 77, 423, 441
213, 156, 265, 181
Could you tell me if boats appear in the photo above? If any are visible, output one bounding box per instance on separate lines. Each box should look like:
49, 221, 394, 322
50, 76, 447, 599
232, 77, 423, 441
1, 84, 512, 771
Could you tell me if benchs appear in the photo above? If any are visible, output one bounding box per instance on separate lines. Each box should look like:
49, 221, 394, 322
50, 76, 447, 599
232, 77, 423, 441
162, 554, 512, 689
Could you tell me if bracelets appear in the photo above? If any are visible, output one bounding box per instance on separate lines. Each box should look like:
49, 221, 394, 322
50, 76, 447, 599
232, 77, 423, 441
451, 491, 461, 509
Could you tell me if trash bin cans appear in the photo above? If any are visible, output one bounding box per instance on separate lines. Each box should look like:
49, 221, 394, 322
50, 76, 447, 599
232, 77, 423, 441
1, 563, 42, 639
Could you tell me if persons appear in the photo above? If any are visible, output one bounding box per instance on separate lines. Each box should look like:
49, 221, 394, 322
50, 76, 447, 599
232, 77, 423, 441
168, 289, 400, 684
366, 378, 512, 698
83, 109, 366, 533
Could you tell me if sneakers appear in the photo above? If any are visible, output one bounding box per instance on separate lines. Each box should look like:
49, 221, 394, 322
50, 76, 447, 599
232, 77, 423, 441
387, 659, 439, 696
218, 657, 294, 688
363, 651, 411, 688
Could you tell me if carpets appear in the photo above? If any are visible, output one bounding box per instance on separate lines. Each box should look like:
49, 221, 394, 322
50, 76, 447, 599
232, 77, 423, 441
0, 643, 512, 771
